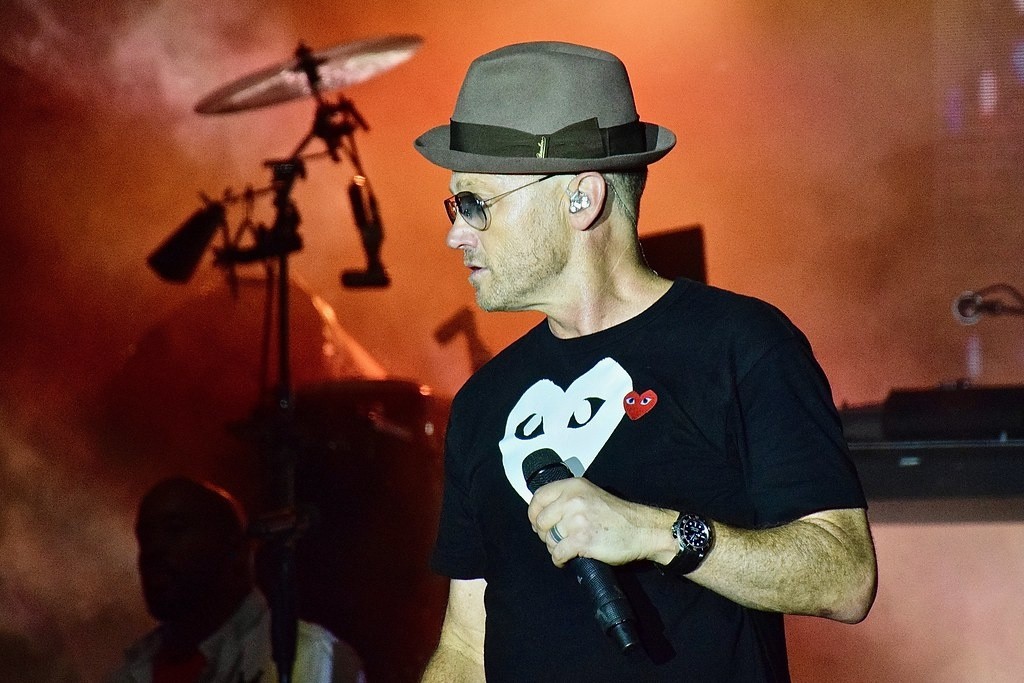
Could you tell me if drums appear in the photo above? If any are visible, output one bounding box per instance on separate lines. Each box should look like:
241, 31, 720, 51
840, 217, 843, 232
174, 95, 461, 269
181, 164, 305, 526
98, 268, 390, 499
224, 374, 457, 683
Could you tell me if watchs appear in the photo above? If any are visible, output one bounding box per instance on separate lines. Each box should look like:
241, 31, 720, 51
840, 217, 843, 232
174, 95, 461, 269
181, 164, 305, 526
653, 509, 713, 578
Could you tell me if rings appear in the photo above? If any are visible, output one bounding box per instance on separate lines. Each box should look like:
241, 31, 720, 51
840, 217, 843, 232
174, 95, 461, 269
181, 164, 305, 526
550, 525, 563, 543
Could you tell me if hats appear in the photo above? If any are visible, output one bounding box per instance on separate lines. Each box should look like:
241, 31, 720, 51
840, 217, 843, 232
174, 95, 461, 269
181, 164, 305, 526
413, 42, 678, 173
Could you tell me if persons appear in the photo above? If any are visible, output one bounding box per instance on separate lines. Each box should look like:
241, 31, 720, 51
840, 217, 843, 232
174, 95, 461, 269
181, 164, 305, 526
415, 41, 879, 683
105, 474, 364, 683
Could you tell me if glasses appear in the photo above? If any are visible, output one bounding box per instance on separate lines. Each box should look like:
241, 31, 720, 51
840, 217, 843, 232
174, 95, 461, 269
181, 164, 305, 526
443, 172, 559, 230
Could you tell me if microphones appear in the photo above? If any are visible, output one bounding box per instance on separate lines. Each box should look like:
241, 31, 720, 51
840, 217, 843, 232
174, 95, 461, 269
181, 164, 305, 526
521, 448, 639, 653
956, 288, 985, 319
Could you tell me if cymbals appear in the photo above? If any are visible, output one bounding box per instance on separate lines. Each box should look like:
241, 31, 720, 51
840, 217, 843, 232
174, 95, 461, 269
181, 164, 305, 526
190, 30, 425, 119
